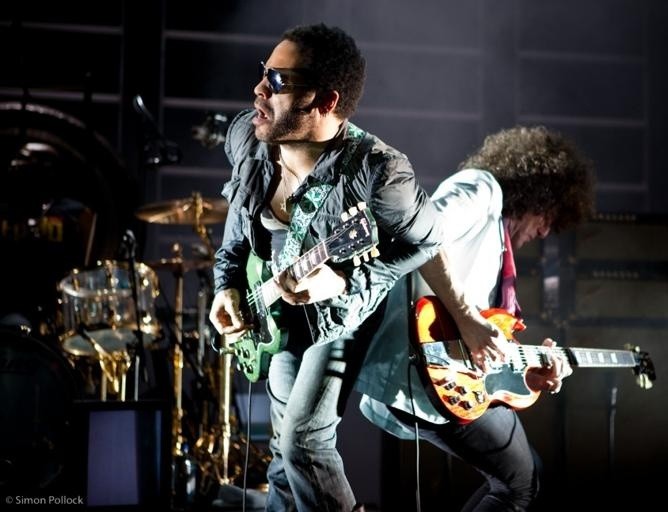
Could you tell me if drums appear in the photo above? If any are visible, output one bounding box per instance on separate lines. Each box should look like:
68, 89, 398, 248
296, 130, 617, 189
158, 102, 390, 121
2, 324, 85, 497
58, 257, 159, 361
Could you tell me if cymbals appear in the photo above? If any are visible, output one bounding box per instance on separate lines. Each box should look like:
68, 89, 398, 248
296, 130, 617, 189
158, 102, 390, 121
145, 258, 214, 271
131, 196, 230, 225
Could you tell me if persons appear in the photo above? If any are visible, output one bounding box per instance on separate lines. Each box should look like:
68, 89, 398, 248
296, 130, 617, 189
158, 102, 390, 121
351, 122, 596, 512
209, 19, 445, 512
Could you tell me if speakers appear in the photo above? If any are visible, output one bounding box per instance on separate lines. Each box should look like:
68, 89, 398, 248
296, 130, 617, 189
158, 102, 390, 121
83, 409, 163, 512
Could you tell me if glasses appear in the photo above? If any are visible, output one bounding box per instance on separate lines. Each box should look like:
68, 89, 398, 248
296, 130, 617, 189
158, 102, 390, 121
260, 60, 309, 95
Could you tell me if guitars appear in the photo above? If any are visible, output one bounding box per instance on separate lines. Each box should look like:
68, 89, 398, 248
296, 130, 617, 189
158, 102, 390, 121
234, 202, 381, 383
409, 295, 656, 425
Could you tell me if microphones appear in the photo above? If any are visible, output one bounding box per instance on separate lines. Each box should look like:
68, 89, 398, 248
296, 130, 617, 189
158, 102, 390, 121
119, 228, 139, 259
132, 95, 166, 140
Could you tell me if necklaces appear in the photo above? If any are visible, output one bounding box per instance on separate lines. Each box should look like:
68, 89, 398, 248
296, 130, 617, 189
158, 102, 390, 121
278, 147, 288, 216
